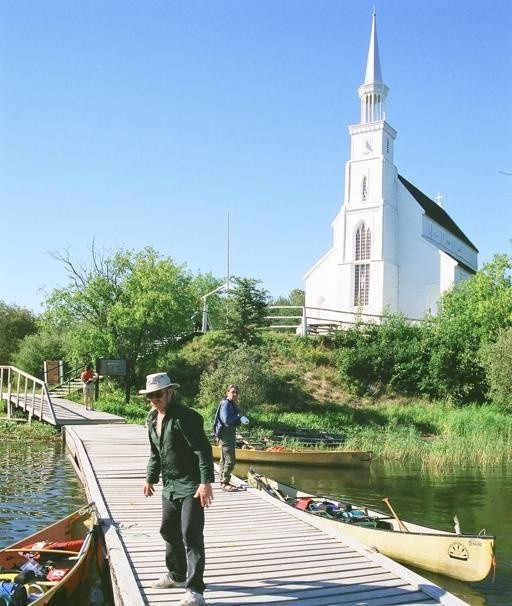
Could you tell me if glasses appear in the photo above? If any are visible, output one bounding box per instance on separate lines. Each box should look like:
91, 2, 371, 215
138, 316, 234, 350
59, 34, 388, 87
146, 391, 166, 398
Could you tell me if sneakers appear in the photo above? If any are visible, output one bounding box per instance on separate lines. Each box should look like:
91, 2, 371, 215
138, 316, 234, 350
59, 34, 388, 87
180, 591, 205, 606
152, 574, 186, 589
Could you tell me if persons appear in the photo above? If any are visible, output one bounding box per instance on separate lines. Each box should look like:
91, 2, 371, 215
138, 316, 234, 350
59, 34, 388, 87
213, 384, 250, 492
81, 361, 99, 411
138, 372, 215, 606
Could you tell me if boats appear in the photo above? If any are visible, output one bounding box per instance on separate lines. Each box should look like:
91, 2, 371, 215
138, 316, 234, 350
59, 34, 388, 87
247, 466, 497, 583
210, 444, 374, 467
0, 500, 99, 605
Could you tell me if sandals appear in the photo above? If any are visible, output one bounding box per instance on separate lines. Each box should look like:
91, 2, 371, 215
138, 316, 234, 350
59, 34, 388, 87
221, 483, 238, 492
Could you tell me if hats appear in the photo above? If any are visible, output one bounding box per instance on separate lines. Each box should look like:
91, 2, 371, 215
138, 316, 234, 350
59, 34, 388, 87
139, 373, 181, 395
